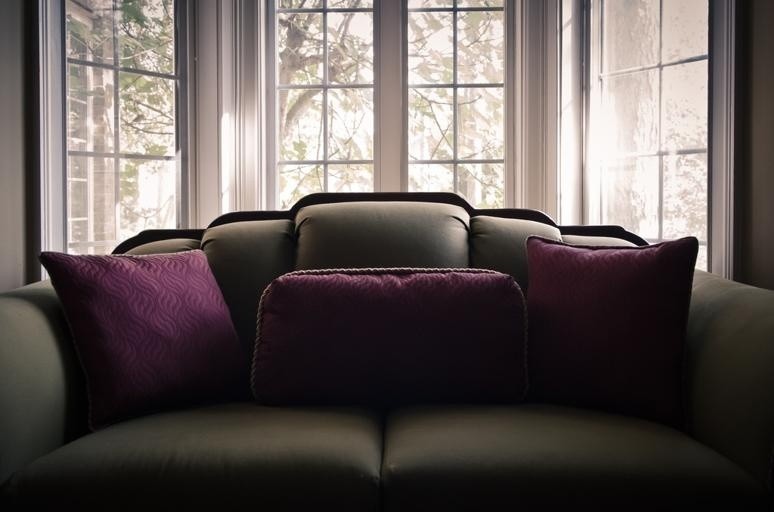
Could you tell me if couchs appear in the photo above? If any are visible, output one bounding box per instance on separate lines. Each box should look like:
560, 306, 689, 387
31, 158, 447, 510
0, 192, 774, 512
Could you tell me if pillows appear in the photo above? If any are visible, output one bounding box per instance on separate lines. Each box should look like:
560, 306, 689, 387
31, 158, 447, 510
527, 236, 699, 425
38, 249, 247, 427
250, 268, 527, 404
